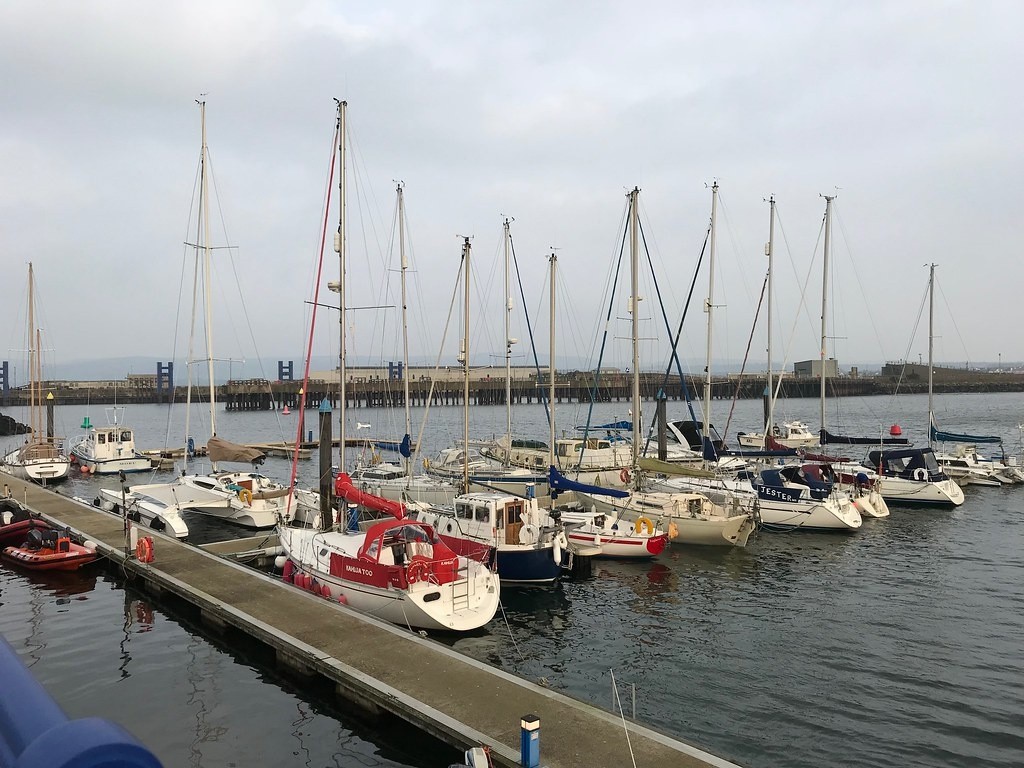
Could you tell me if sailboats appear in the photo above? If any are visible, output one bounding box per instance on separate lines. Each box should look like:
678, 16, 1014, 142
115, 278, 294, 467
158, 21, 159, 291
96, 94, 1024, 634
2, 261, 74, 490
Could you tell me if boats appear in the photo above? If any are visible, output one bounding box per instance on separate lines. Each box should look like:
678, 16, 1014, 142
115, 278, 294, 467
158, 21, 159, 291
70, 379, 153, 476
0, 498, 51, 541
0, 525, 98, 572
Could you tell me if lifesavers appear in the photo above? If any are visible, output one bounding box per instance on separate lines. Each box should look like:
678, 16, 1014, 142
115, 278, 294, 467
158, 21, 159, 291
136, 537, 150, 562
635, 517, 653, 534
519, 523, 538, 544
409, 561, 430, 583
620, 469, 628, 482
913, 467, 928, 480
239, 488, 252, 502
